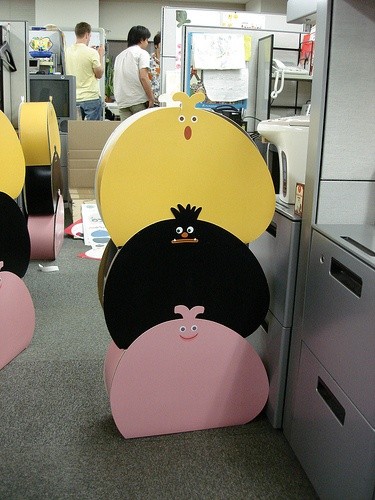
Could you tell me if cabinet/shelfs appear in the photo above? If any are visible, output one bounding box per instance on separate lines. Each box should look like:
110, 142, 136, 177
245, 193, 303, 432
285, 0, 317, 30
286, 221, 374, 500
252, 33, 318, 168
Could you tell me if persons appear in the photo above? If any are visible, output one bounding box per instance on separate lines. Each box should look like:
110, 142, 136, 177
147, 32, 161, 108
112, 26, 154, 121
65, 22, 106, 121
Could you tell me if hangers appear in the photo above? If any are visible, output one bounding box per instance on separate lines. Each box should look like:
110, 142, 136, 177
0, 23, 17, 74
